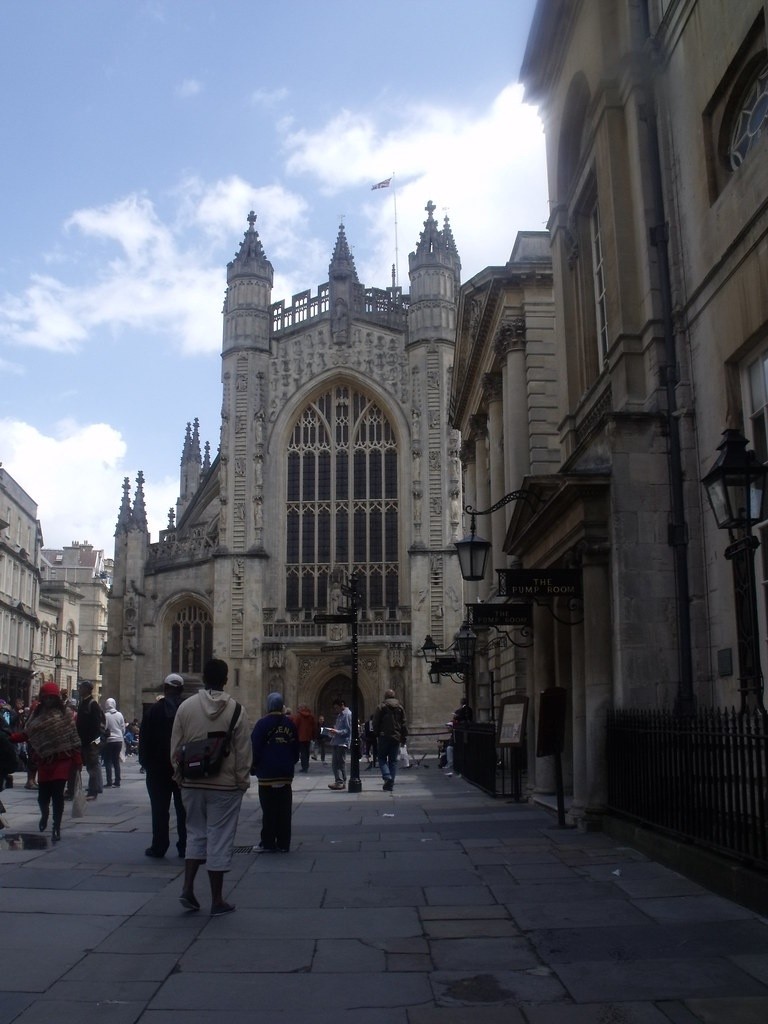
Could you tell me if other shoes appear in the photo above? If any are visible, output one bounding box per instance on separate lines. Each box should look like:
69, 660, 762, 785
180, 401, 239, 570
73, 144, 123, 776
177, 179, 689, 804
210, 903, 237, 916
113, 782, 120, 787
104, 784, 112, 788
52, 829, 60, 841
383, 779, 393, 792
312, 755, 317, 761
279, 848, 288, 853
145, 848, 166, 858
178, 894, 200, 910
252, 845, 272, 853
178, 851, 206, 864
24, 781, 39, 790
39, 817, 47, 832
86, 792, 97, 800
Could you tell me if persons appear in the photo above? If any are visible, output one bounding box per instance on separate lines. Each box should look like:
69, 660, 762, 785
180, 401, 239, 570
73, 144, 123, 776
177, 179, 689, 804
64, 682, 108, 801
138, 673, 187, 857
311, 715, 328, 761
328, 699, 352, 790
170, 659, 253, 916
373, 688, 408, 791
125, 718, 138, 740
0, 696, 40, 792
249, 692, 300, 852
442, 699, 474, 769
285, 704, 317, 773
61, 695, 78, 720
365, 714, 378, 768
101, 698, 126, 789
9, 682, 83, 842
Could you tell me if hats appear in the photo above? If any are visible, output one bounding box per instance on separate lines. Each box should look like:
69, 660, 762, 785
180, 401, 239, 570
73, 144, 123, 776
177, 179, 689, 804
156, 695, 165, 701
78, 680, 94, 691
446, 721, 453, 727
39, 682, 60, 697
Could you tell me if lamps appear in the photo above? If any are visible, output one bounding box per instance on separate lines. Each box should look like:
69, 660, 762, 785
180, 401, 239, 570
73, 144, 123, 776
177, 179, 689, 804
421, 625, 507, 683
454, 489, 540, 581
30, 650, 63, 668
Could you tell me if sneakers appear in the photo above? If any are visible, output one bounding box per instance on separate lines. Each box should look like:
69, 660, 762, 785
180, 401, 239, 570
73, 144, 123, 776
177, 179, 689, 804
328, 783, 346, 790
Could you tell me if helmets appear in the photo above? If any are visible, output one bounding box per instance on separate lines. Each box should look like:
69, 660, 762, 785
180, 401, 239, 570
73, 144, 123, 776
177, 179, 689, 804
266, 693, 283, 713
164, 673, 184, 687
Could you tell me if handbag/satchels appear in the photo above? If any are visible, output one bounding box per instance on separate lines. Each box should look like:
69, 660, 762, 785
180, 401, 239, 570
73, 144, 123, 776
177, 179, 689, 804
119, 740, 126, 763
71, 770, 89, 818
0, 729, 17, 774
400, 745, 410, 768
174, 735, 229, 779
